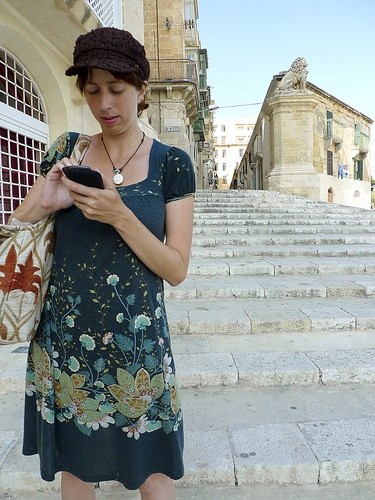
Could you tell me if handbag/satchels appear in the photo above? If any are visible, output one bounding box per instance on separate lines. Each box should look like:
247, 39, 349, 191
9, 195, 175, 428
0, 132, 92, 345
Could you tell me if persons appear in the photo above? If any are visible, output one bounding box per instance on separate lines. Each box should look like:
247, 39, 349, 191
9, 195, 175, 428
7, 27, 196, 500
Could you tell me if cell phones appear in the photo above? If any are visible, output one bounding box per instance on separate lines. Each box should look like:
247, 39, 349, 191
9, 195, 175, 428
62, 165, 105, 189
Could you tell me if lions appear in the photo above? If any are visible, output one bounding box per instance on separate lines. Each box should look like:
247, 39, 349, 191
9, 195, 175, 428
275, 57, 308, 92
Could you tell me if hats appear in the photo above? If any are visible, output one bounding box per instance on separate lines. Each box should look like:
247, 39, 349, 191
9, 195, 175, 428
65, 27, 150, 82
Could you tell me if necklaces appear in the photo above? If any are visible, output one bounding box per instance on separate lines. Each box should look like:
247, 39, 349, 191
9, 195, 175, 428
101, 131, 145, 185
